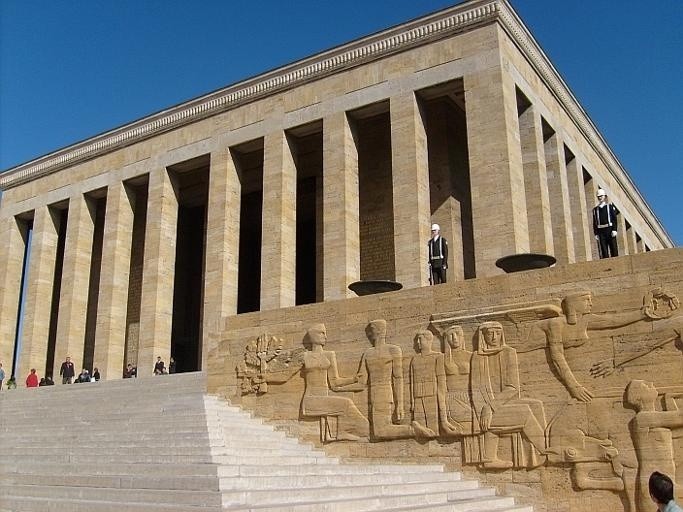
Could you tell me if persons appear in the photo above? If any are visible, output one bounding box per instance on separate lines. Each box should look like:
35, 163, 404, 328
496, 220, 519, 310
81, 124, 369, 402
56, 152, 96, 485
592, 189, 619, 259
251, 294, 683, 470
427, 223, 448, 285
0, 354, 176, 391
626, 380, 682, 512
648, 471, 683, 512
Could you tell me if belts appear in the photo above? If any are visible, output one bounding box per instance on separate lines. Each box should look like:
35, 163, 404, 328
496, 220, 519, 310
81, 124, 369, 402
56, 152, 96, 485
430, 256, 443, 260
597, 223, 613, 228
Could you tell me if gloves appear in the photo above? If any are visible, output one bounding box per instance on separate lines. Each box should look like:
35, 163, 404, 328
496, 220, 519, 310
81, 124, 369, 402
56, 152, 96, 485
611, 231, 617, 237
594, 235, 599, 241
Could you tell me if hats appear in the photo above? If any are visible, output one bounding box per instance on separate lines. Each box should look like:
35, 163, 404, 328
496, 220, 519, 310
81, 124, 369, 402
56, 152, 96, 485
431, 224, 441, 231
596, 189, 605, 196
